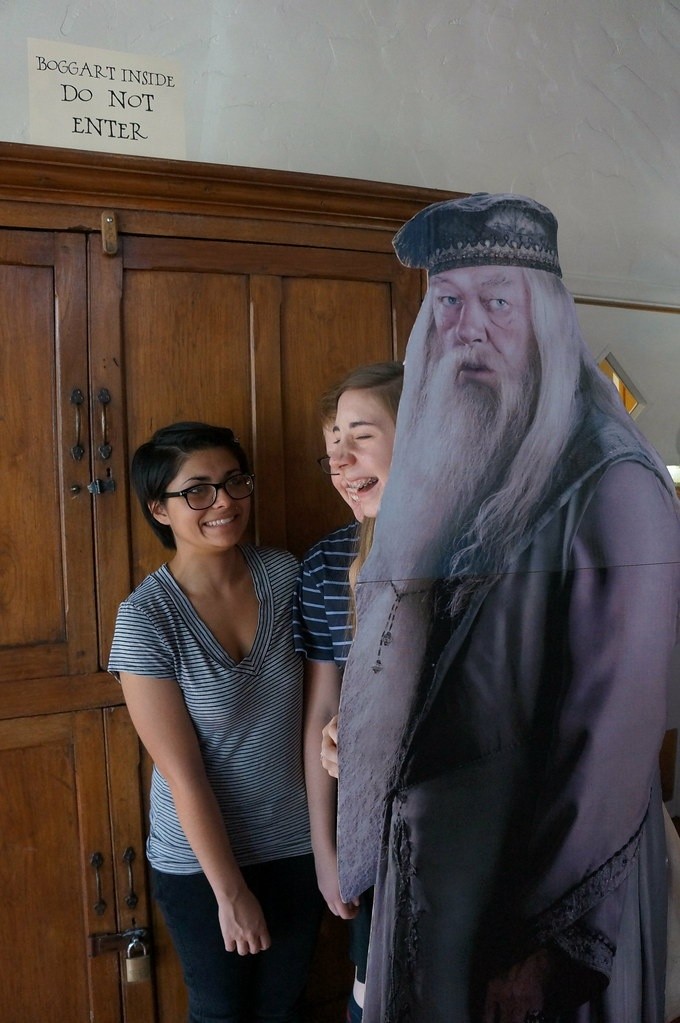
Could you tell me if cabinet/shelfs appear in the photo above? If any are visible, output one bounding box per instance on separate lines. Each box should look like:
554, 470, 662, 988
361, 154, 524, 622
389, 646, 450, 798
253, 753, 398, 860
0, 141, 488, 1022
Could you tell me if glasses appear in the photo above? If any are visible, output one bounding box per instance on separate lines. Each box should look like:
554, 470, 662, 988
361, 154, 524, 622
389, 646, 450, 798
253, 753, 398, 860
160, 471, 255, 510
318, 454, 340, 475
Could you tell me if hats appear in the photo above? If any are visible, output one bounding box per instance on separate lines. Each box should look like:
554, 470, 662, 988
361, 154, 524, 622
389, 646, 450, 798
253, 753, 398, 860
390, 189, 563, 279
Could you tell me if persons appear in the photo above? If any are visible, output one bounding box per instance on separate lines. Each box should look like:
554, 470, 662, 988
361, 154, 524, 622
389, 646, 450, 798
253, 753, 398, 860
294, 385, 370, 921
327, 190, 680, 1023
318, 364, 411, 774
108, 422, 307, 1021
